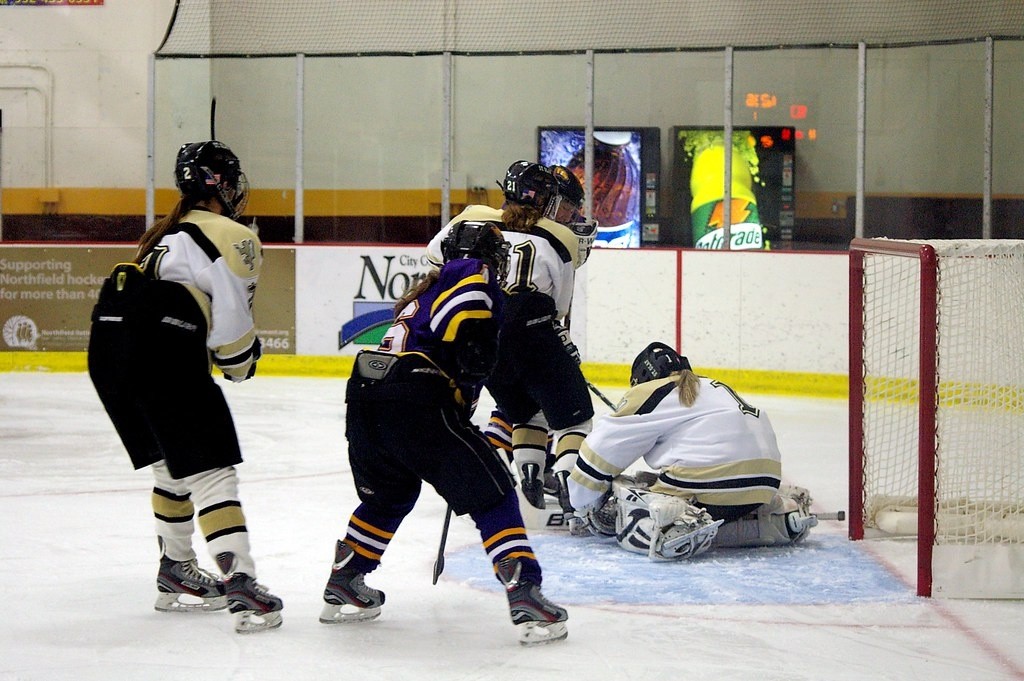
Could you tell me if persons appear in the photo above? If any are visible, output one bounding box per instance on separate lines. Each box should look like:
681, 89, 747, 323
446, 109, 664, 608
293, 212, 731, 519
318, 160, 781, 626
87, 141, 284, 615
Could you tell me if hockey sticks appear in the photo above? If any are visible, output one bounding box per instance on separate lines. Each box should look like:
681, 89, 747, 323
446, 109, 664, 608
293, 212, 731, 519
583, 376, 617, 411
496, 447, 845, 529
433, 385, 475, 585
564, 270, 575, 333
211, 97, 216, 140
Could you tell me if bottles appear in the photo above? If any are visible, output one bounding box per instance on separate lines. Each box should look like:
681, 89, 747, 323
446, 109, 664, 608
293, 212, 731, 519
686, 131, 764, 250
564, 131, 639, 250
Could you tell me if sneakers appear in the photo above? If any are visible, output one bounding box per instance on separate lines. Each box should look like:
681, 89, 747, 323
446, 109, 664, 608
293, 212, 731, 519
214, 551, 283, 635
521, 462, 547, 510
320, 540, 386, 624
498, 558, 568, 647
554, 470, 576, 519
154, 535, 227, 612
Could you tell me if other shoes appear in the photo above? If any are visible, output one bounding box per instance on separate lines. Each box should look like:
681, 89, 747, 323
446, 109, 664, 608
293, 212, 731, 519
543, 473, 558, 495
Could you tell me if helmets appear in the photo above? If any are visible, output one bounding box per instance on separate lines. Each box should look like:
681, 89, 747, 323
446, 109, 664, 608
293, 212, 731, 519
441, 220, 512, 288
496, 160, 559, 221
630, 342, 693, 387
175, 140, 250, 219
550, 164, 586, 229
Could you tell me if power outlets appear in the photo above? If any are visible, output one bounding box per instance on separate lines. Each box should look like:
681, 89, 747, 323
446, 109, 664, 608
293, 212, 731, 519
831, 202, 839, 214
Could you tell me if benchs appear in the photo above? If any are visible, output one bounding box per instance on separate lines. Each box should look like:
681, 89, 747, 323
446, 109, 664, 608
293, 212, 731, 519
847, 196, 1024, 242
